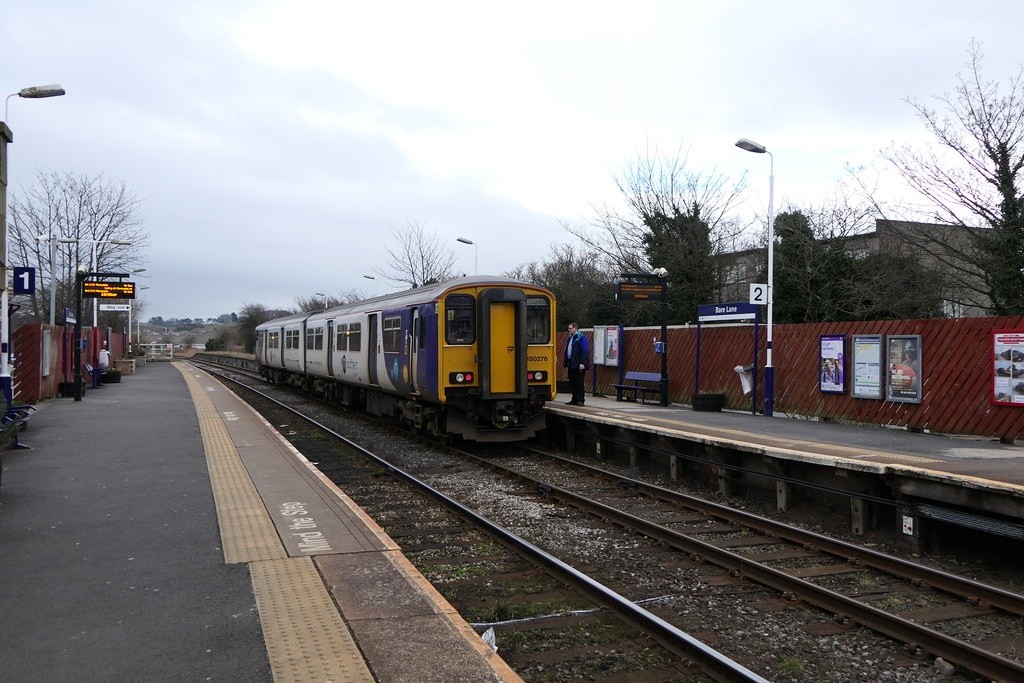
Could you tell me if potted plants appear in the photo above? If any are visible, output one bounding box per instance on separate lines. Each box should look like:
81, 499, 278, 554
114, 355, 136, 375
103, 366, 121, 383
132, 347, 146, 366
690, 389, 728, 412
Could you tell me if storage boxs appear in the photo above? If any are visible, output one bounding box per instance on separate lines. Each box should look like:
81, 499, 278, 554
60, 378, 86, 396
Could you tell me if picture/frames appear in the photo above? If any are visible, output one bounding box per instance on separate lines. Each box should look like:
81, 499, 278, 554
819, 334, 848, 392
991, 329, 1024, 406
886, 335, 922, 404
851, 334, 884, 401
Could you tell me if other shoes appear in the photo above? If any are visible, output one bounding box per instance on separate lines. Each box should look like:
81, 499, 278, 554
565, 400, 584, 406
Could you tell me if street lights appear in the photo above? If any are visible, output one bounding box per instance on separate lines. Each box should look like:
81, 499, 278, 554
129, 269, 146, 357
316, 293, 327, 311
736, 139, 773, 416
0, 85, 65, 451
50, 233, 131, 326
457, 237, 477, 275
137, 287, 150, 349
363, 276, 379, 297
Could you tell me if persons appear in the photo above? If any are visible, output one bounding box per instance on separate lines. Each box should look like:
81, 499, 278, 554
564, 322, 590, 406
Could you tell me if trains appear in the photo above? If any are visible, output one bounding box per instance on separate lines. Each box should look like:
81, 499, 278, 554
255, 275, 556, 449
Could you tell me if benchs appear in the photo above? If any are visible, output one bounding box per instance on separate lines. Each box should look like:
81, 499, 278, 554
608, 372, 661, 404
85, 363, 107, 389
